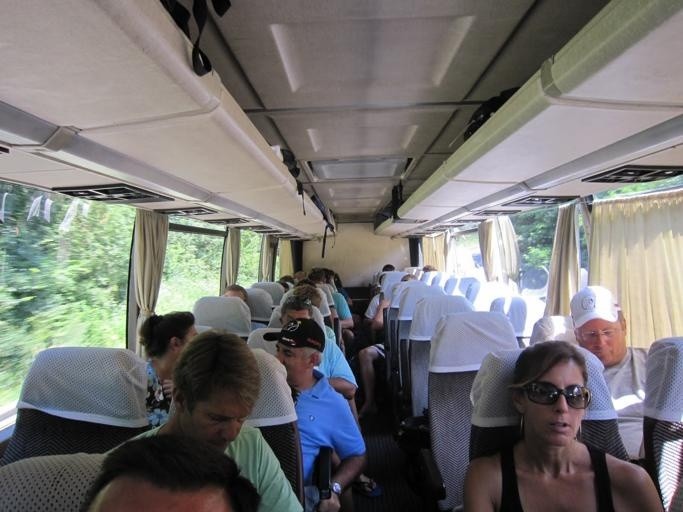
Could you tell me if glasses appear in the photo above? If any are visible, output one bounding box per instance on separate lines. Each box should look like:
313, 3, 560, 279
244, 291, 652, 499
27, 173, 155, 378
516, 381, 590, 408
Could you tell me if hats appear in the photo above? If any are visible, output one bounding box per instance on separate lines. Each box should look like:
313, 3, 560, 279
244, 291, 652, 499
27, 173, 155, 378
262, 319, 323, 352
570, 286, 622, 327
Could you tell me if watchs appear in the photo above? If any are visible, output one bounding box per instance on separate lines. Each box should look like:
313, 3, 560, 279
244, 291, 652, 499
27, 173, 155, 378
331, 481, 343, 497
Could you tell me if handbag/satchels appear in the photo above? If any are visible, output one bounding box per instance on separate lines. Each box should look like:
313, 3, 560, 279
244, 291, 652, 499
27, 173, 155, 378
463, 87, 519, 139
373, 199, 406, 228
310, 194, 326, 220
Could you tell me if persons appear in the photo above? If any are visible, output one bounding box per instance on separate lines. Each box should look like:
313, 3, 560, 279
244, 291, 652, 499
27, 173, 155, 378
138, 311, 198, 428
571, 284, 651, 458
276, 265, 438, 419
264, 318, 370, 512
279, 293, 383, 512
464, 342, 665, 511
224, 285, 268, 332
103, 334, 303, 511
80, 432, 263, 512
